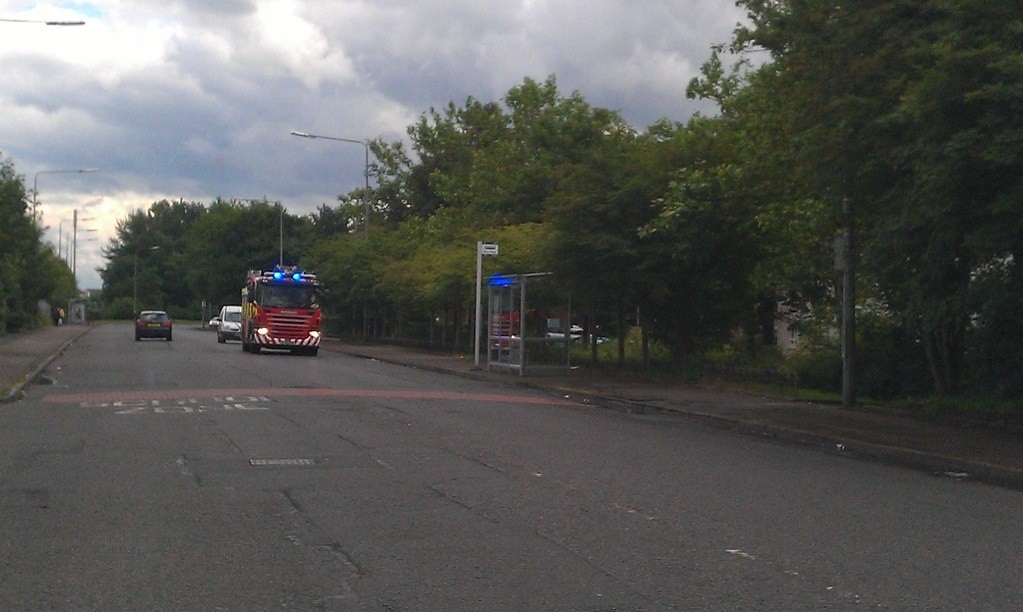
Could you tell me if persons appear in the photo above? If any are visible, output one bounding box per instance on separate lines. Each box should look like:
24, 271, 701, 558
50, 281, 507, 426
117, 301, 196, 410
52, 305, 65, 325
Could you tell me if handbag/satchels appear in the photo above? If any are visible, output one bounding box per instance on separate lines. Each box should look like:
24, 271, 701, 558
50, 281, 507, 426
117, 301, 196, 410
58, 318, 62, 326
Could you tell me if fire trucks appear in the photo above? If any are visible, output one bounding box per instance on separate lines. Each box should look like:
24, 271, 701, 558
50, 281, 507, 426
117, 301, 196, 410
239, 263, 327, 356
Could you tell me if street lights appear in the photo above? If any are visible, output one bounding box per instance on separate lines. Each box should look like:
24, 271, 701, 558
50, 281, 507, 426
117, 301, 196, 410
57, 216, 99, 322
233, 196, 284, 266
291, 131, 370, 346
30, 168, 99, 330
134, 245, 160, 320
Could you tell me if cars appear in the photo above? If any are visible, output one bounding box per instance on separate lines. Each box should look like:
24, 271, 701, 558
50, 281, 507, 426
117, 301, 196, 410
208, 316, 219, 328
216, 303, 243, 343
135, 310, 172, 341
